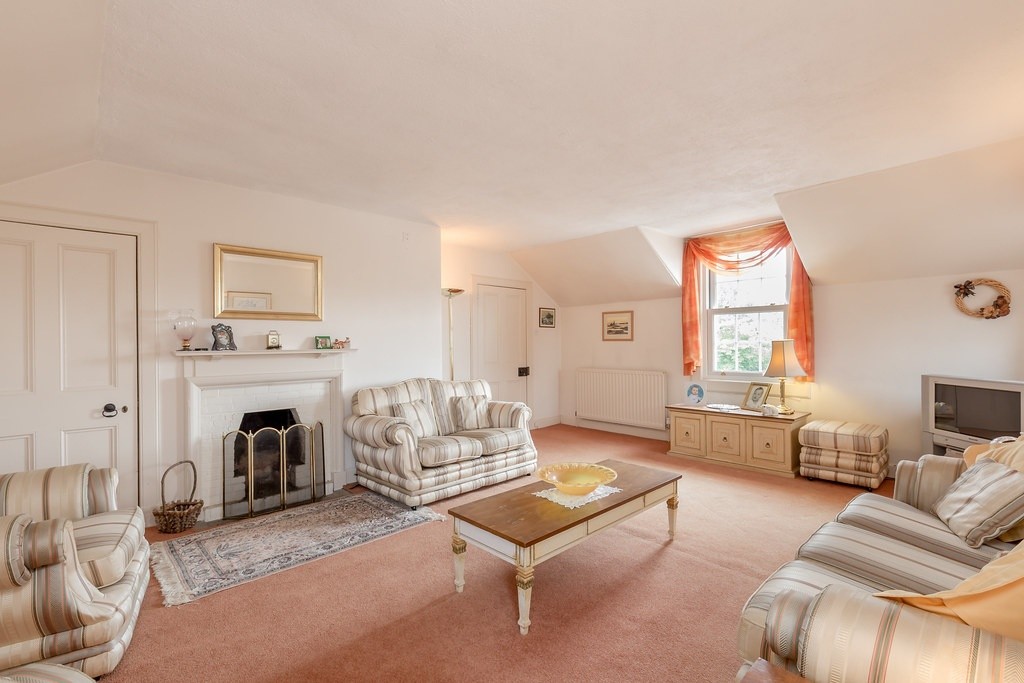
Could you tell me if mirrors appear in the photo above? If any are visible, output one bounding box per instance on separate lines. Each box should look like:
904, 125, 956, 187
212, 242, 324, 321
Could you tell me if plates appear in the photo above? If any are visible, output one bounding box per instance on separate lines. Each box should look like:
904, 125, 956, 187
707, 404, 740, 409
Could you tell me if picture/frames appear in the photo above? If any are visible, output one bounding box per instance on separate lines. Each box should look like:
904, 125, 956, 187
539, 308, 556, 328
226, 291, 272, 310
210, 323, 237, 351
740, 382, 773, 412
602, 311, 634, 342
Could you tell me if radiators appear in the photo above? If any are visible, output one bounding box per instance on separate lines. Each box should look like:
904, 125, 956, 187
575, 367, 670, 431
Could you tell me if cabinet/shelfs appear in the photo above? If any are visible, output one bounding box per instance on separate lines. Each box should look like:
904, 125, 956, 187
666, 403, 811, 479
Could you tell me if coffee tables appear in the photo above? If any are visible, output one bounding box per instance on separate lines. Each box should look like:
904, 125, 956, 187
447, 459, 683, 636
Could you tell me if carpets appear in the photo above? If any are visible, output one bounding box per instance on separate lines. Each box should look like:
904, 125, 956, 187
149, 489, 447, 608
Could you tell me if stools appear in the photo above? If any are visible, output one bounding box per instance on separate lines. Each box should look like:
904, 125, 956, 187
797, 419, 889, 492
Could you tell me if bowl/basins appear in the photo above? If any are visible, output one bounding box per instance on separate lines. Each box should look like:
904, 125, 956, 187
537, 462, 618, 495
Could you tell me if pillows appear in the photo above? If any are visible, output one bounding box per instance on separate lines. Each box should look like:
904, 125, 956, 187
392, 398, 438, 438
929, 456, 1024, 549
451, 394, 493, 431
961, 432, 1024, 542
870, 538, 1024, 640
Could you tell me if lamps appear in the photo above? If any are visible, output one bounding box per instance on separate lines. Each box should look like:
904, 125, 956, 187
763, 339, 808, 415
442, 287, 464, 380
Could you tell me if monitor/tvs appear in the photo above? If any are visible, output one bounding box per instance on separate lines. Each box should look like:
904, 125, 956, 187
928, 377, 1024, 450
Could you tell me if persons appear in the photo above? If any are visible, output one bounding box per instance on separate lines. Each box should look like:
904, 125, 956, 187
688, 386, 700, 402
746, 387, 764, 407
218, 331, 227, 345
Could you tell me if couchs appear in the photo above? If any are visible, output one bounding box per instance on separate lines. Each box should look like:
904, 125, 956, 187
342, 378, 539, 512
0, 463, 152, 677
735, 454, 1024, 683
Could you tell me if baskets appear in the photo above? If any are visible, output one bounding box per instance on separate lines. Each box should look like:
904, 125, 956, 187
153, 460, 204, 533
